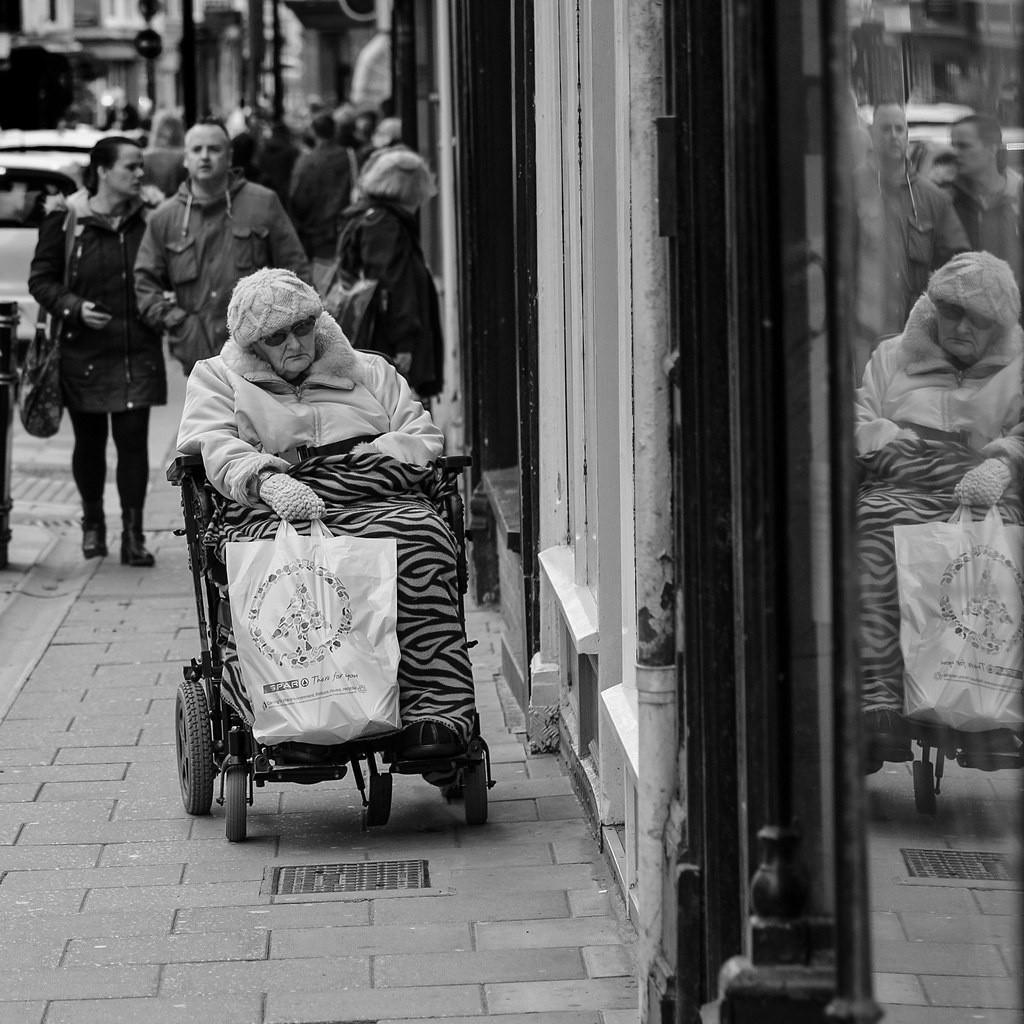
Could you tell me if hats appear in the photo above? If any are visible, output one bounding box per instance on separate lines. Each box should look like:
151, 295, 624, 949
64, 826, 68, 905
226, 267, 324, 347
927, 251, 1021, 329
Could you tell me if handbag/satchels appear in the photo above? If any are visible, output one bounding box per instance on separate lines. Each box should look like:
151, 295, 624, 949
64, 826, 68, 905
892, 505, 1024, 733
316, 235, 408, 349
18, 330, 63, 439
226, 518, 402, 745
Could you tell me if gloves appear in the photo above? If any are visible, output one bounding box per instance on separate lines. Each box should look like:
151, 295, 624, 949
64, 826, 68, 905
349, 441, 380, 456
953, 459, 1012, 507
260, 473, 327, 523
894, 428, 920, 441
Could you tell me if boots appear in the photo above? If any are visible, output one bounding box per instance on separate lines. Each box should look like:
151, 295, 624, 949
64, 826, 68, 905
81, 498, 107, 558
121, 508, 154, 566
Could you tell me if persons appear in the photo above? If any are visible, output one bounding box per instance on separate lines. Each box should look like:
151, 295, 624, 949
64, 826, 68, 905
29, 136, 177, 566
939, 116, 1024, 290
853, 102, 971, 387
856, 253, 1023, 767
133, 117, 315, 373
340, 145, 447, 411
232, 98, 401, 259
174, 268, 476, 783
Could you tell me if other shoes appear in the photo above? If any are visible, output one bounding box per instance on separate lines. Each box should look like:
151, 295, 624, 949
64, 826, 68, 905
279, 741, 323, 765
864, 710, 902, 744
987, 730, 1015, 752
400, 720, 456, 757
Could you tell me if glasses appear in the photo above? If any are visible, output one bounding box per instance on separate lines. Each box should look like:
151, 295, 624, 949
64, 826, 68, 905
935, 301, 994, 331
260, 316, 315, 346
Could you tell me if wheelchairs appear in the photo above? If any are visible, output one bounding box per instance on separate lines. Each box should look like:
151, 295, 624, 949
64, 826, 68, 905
839, 448, 1024, 817
165, 455, 499, 842
